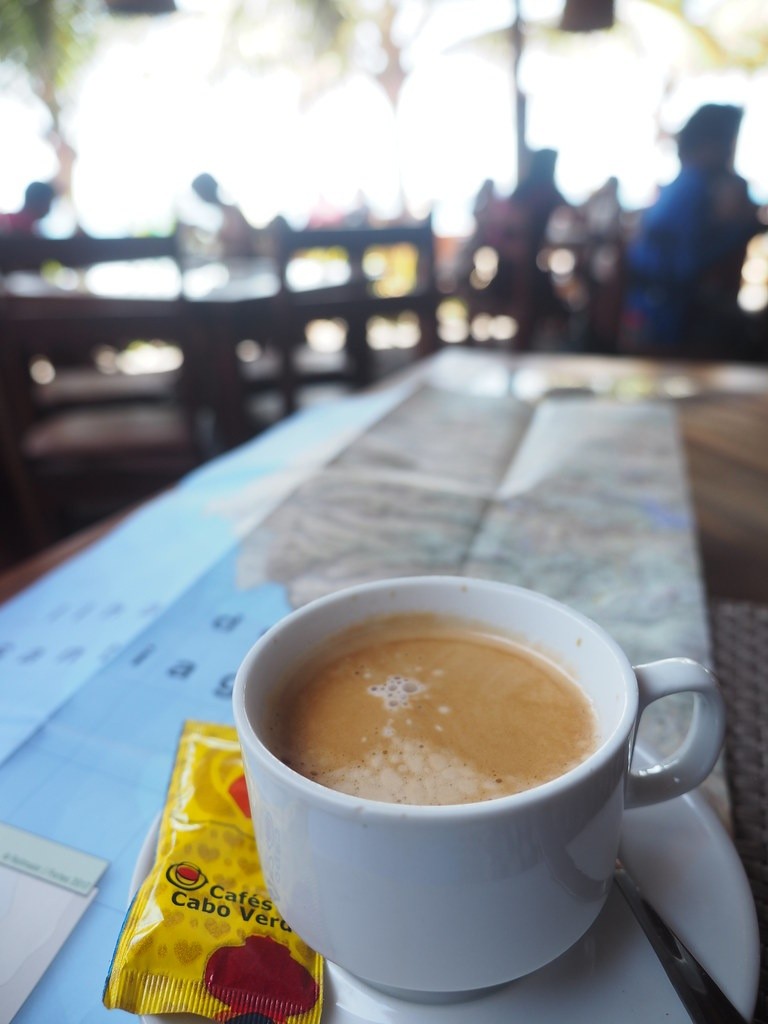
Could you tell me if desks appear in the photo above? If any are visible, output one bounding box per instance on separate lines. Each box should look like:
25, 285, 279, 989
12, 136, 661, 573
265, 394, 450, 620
0, 261, 375, 564
0, 349, 768, 1024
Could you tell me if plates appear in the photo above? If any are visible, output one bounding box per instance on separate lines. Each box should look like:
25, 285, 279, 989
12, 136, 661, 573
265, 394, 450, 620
127, 742, 760, 1024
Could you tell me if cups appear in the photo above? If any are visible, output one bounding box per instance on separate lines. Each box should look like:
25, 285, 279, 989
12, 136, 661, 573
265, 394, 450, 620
231, 575, 726, 993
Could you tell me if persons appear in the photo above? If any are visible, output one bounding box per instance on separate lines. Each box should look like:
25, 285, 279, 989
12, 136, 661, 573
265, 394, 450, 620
448, 92, 757, 359
0, 172, 255, 282
